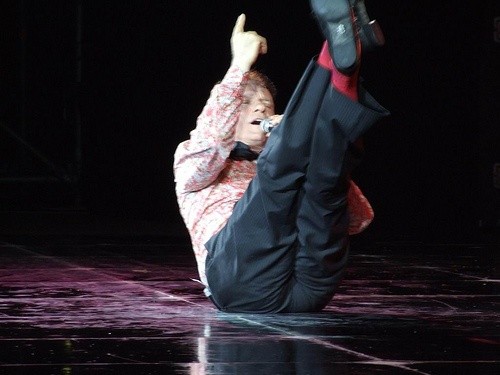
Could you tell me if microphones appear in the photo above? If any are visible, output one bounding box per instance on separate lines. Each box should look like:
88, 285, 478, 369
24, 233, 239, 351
259, 119, 279, 132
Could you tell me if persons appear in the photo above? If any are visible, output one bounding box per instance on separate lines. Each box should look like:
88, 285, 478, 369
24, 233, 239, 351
173, 0, 385, 315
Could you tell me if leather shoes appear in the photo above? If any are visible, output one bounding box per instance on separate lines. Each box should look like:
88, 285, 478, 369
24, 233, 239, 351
309, 0, 385, 75
